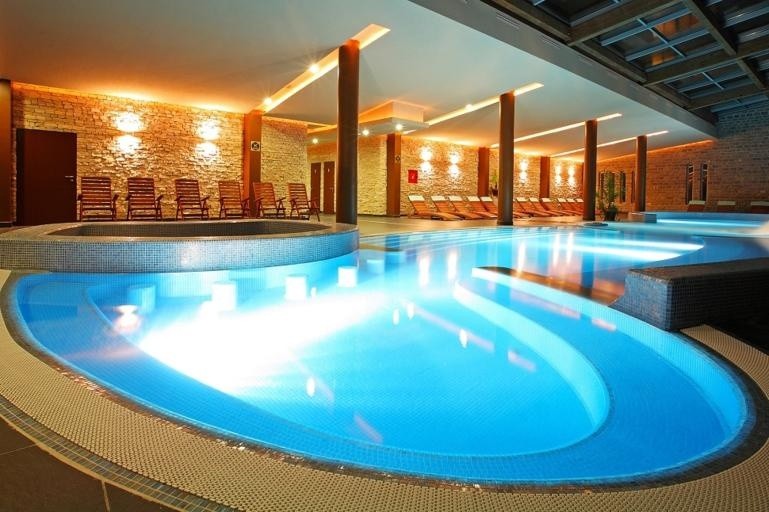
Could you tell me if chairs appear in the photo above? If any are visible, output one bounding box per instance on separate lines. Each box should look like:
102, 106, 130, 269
513, 197, 584, 218
217, 181, 251, 219
125, 178, 164, 221
78, 176, 120, 221
174, 179, 210, 221
288, 183, 320, 221
253, 182, 287, 219
408, 195, 498, 221
